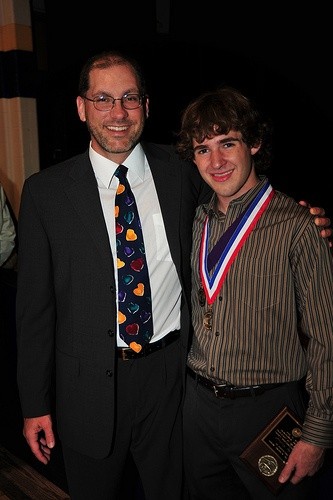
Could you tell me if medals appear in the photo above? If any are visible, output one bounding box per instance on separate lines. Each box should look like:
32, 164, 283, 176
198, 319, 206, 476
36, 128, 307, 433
198, 289, 206, 307
203, 309, 213, 330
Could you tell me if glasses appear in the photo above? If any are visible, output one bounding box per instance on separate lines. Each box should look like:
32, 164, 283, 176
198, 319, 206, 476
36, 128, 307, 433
84, 94, 144, 111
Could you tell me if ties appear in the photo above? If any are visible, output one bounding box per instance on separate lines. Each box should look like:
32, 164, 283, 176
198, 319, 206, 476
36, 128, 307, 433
114, 164, 154, 354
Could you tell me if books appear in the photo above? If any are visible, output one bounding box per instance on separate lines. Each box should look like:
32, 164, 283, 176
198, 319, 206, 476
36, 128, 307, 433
239, 406, 304, 497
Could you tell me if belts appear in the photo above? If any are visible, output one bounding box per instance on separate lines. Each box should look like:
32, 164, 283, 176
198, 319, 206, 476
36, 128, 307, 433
115, 329, 181, 361
185, 365, 282, 400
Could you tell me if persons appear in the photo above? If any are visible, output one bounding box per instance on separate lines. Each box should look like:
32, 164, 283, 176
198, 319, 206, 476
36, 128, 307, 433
174, 89, 333, 500
0, 184, 17, 268
16, 49, 333, 500
